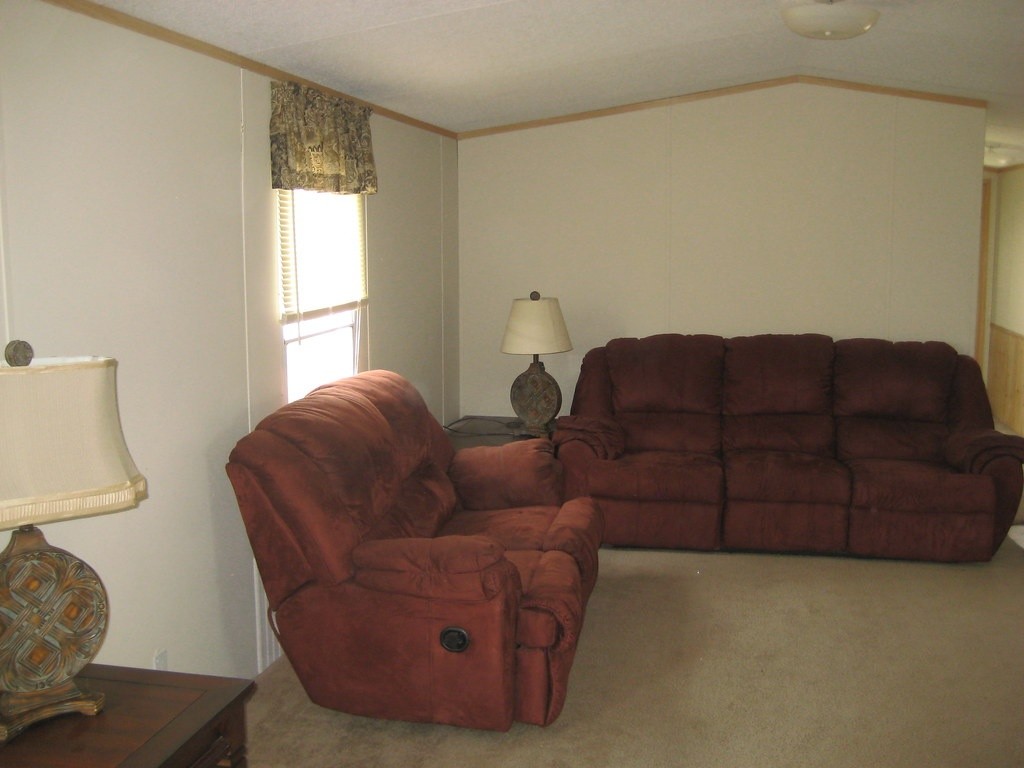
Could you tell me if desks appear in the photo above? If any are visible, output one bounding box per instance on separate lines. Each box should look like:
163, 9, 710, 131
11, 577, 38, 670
0, 662, 259, 768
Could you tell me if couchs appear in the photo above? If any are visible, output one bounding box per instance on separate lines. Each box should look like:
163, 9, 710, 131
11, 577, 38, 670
560, 332, 1024, 564
226, 370, 603, 733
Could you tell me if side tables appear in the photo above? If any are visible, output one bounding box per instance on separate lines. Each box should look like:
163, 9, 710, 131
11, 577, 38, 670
447, 416, 526, 451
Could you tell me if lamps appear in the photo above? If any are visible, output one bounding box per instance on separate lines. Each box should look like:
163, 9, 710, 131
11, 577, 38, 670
782, 0, 880, 40
501, 291, 573, 439
0, 341, 149, 746
